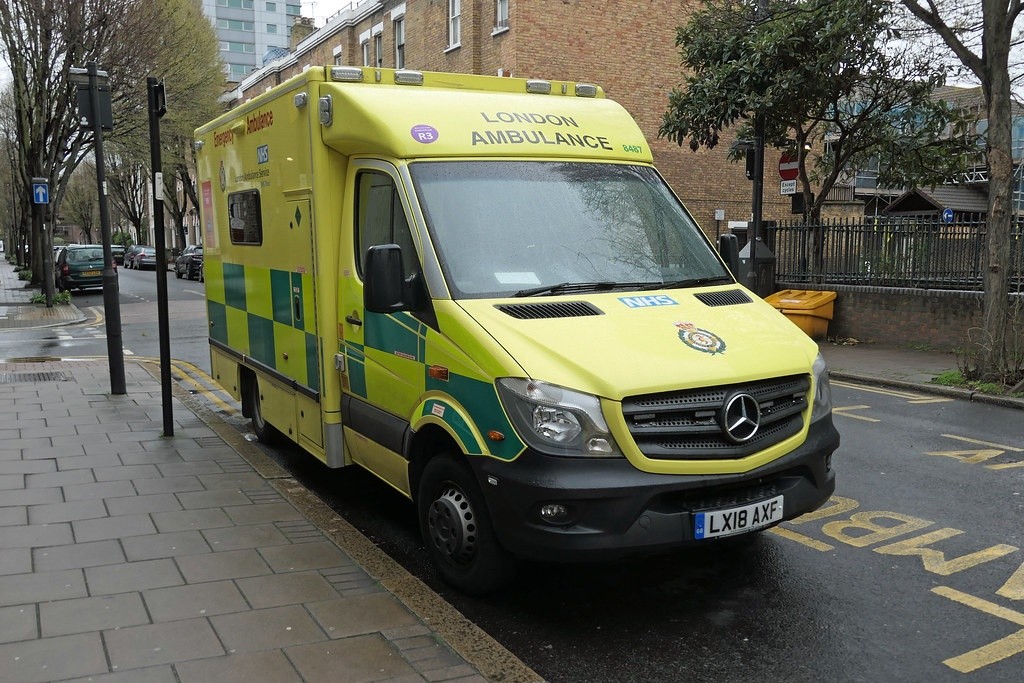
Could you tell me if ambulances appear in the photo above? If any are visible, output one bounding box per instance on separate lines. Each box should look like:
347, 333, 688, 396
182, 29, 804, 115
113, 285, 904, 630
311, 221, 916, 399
193, 62, 841, 600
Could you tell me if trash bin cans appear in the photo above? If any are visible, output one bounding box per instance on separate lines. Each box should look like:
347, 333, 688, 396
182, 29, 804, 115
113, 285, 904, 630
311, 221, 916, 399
760, 288, 837, 341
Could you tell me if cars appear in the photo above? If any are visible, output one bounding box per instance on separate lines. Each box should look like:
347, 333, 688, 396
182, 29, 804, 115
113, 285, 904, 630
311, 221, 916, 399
53, 244, 120, 294
123, 244, 156, 271
111, 244, 126, 266
173, 244, 204, 281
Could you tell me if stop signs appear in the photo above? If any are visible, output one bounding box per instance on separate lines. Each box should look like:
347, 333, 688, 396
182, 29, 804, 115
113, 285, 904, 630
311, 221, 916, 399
779, 149, 799, 181
